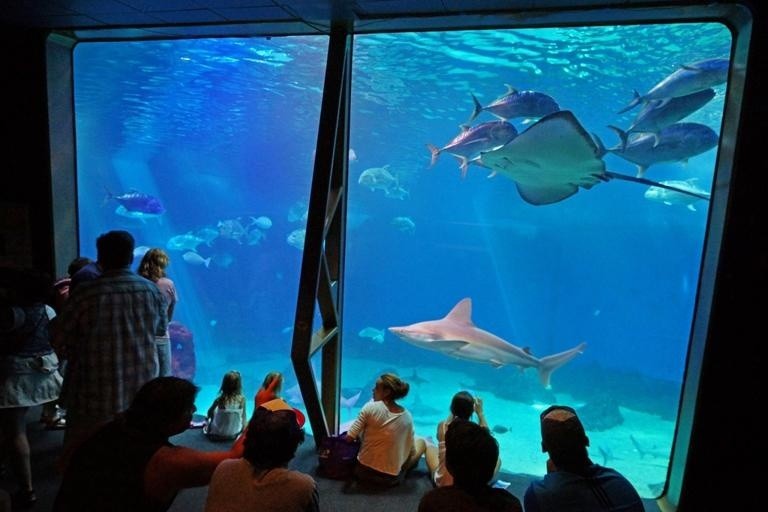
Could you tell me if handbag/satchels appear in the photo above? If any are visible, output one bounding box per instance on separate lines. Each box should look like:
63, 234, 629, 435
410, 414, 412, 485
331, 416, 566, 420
478, 413, 482, 417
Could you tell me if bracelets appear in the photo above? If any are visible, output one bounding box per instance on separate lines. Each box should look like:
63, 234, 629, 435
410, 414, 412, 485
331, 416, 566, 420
478, 413, 482, 417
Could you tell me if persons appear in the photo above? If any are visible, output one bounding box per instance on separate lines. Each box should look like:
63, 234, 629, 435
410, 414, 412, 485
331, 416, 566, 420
46, 231, 167, 453
42, 375, 279, 509
137, 248, 177, 376
344, 373, 426, 489
256, 374, 282, 411
4, 267, 65, 504
424, 391, 501, 488
51, 279, 71, 429
204, 398, 318, 511
523, 405, 645, 512
417, 419, 523, 512
189, 372, 246, 440
67, 257, 93, 279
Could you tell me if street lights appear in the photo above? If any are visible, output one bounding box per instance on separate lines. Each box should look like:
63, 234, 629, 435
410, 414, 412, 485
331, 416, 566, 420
317, 430, 362, 481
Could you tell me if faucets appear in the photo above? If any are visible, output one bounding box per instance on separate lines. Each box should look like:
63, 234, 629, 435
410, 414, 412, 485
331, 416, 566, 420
40, 412, 67, 429
9, 488, 37, 505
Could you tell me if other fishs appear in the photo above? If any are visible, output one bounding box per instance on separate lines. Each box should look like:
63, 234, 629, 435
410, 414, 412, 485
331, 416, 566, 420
644, 178, 712, 210
590, 121, 721, 179
390, 216, 415, 230
617, 57, 729, 114
608, 89, 716, 154
467, 84, 559, 124
433, 120, 517, 172
466, 110, 711, 203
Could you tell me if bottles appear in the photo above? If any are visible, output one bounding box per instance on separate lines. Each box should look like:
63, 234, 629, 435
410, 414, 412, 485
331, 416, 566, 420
539, 404, 584, 448
